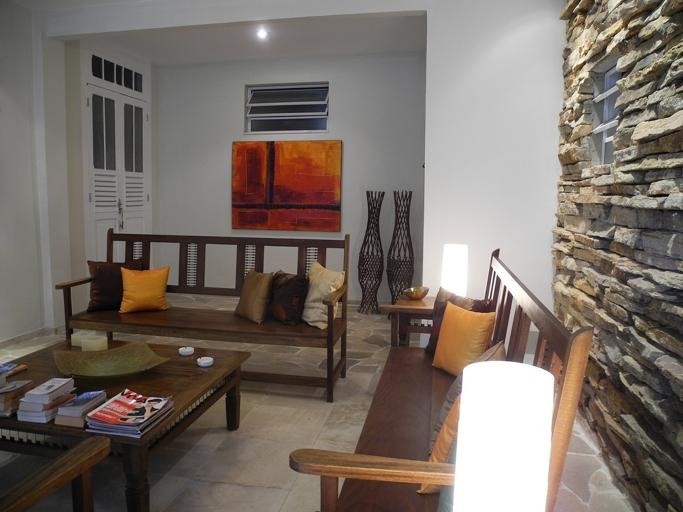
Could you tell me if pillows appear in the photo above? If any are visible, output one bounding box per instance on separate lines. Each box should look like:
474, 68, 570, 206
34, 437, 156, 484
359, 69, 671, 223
423, 288, 497, 357
436, 436, 457, 512
416, 393, 460, 496
269, 269, 309, 328
234, 270, 274, 326
430, 301, 496, 377
119, 266, 171, 313
426, 339, 505, 456
306, 261, 345, 330
87, 256, 149, 313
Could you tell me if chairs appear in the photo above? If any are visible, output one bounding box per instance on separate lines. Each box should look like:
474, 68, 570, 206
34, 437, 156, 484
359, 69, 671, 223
0, 435, 112, 512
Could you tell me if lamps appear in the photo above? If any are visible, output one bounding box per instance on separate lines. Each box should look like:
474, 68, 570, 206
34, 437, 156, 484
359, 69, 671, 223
452, 361, 556, 512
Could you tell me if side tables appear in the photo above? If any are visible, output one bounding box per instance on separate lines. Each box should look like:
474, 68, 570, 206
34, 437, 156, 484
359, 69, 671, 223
377, 292, 439, 348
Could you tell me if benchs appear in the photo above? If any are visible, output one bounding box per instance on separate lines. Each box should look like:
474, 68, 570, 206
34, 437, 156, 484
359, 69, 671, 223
290, 248, 595, 512
56, 226, 350, 407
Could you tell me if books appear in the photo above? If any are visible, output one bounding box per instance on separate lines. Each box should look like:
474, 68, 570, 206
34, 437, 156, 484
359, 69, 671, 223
0, 363, 176, 439
